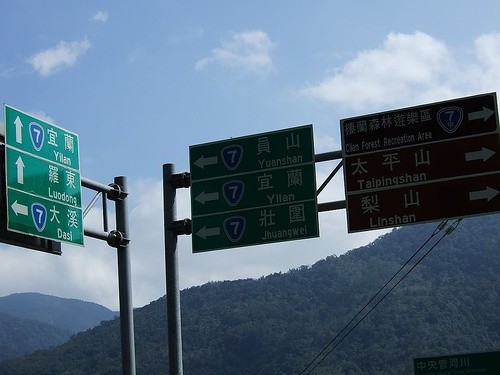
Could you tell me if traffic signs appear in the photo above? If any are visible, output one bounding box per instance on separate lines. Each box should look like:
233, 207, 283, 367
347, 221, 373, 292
341, 92, 499, 234
189, 124, 320, 254
413, 352, 500, 375
4, 103, 85, 246
